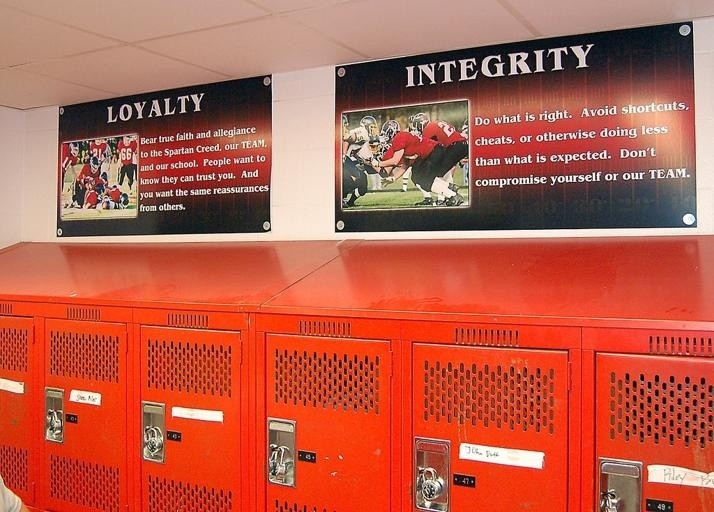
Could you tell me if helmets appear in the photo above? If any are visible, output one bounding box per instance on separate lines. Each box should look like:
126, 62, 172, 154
70, 142, 80, 157
120, 193, 129, 207
361, 116, 399, 152
102, 196, 110, 209
89, 156, 100, 174
409, 113, 429, 132
96, 184, 105, 195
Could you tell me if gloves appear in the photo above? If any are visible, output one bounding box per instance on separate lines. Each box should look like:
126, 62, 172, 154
364, 157, 380, 168
380, 176, 395, 187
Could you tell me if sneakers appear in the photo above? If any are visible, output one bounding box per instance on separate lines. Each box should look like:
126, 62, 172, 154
63, 203, 74, 208
343, 200, 363, 209
414, 183, 465, 206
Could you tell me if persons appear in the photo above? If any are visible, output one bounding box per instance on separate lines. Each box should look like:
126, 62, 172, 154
340, 111, 469, 208
61, 133, 137, 210
